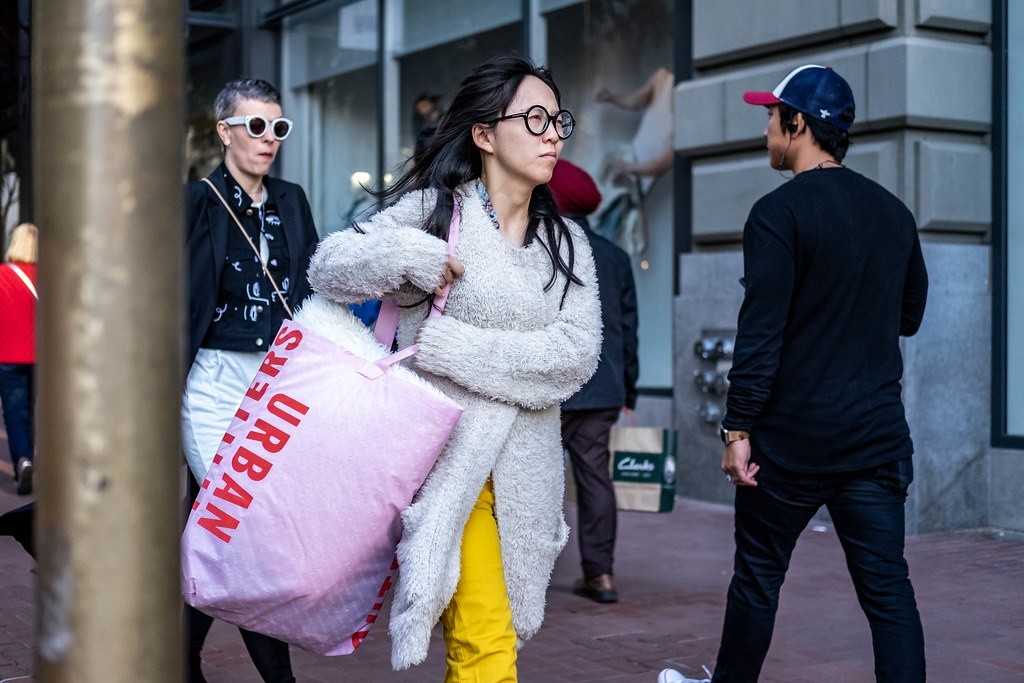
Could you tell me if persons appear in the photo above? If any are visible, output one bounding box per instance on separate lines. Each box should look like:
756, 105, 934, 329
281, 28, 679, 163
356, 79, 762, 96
545, 158, 639, 604
657, 65, 929, 683
178, 78, 320, 683
306, 57, 604, 683
0, 223, 38, 497
594, 58, 690, 261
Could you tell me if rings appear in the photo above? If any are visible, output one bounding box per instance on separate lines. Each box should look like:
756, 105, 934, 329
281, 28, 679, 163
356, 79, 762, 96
733, 476, 740, 485
725, 474, 732, 483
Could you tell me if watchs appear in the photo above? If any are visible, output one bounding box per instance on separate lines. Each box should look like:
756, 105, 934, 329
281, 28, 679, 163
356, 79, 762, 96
720, 428, 749, 446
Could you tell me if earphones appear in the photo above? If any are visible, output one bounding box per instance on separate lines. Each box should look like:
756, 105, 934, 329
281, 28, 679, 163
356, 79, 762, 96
787, 119, 798, 133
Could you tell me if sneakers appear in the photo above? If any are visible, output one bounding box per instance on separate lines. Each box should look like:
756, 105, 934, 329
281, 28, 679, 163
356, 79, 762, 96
657, 668, 713, 682
14, 457, 34, 495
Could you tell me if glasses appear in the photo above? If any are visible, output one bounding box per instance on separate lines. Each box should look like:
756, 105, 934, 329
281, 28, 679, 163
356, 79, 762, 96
224, 116, 295, 141
480, 105, 579, 141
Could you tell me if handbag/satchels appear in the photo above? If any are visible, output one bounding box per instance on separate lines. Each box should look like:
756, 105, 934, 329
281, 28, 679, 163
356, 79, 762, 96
182, 189, 462, 658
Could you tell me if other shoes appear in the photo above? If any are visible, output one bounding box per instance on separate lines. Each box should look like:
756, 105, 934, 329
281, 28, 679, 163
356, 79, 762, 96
575, 577, 620, 602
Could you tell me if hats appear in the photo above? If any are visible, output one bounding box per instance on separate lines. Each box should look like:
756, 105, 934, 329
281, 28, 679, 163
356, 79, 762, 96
743, 63, 856, 131
